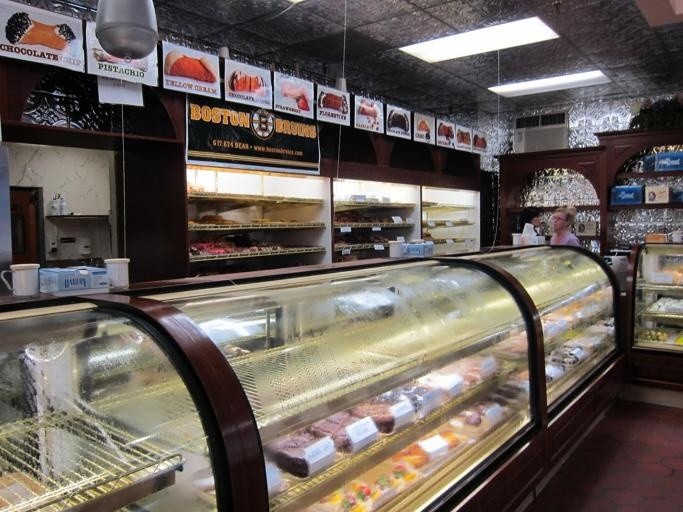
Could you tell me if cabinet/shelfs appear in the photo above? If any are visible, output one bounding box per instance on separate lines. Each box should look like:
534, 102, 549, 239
188, 188, 325, 262
422, 200, 475, 246
593, 126, 683, 255
624, 244, 683, 388
333, 198, 416, 253
0, 247, 624, 512
494, 145, 606, 259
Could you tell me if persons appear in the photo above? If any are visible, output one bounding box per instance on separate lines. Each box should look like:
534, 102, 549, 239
550, 207, 582, 247
530, 216, 540, 226
536, 211, 548, 236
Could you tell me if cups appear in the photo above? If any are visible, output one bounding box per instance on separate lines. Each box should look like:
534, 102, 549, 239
388, 238, 405, 258
511, 222, 545, 246
1, 262, 40, 299
667, 230, 682, 243
104, 258, 131, 290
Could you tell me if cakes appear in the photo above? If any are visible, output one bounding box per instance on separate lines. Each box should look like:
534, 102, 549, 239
262, 290, 615, 512
2, 11, 488, 151
